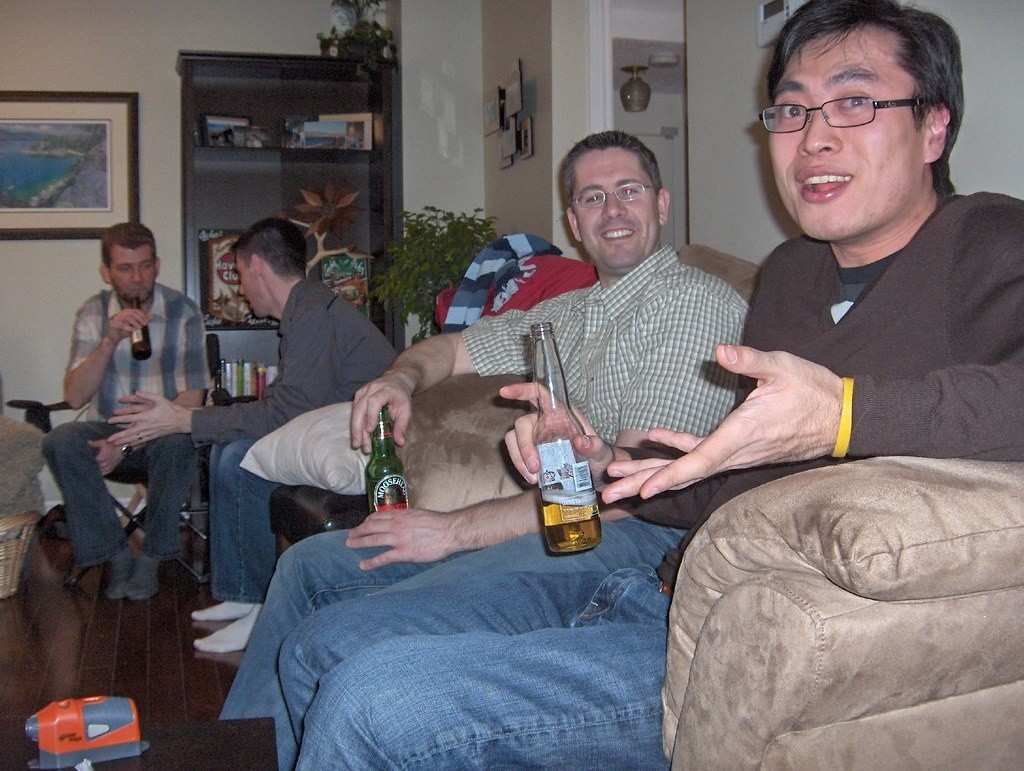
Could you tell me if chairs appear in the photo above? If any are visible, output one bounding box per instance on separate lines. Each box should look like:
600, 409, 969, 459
6, 333, 234, 588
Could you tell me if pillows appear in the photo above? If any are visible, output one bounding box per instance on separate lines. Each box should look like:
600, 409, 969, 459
238, 397, 377, 497
0, 414, 50, 544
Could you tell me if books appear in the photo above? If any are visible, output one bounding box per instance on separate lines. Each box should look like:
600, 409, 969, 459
206, 334, 278, 401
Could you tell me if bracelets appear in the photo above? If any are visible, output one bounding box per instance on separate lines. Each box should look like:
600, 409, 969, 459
832, 378, 854, 457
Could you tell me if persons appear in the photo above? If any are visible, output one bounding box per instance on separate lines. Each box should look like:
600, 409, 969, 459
331, 133, 345, 149
284, 121, 304, 148
346, 127, 359, 149
277, 0, 1024, 771
219, 131, 751, 771
108, 217, 398, 654
223, 127, 262, 148
42, 223, 211, 600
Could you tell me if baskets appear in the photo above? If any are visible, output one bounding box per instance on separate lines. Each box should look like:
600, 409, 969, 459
0, 511, 40, 600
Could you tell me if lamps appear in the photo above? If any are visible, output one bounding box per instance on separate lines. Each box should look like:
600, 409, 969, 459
620, 65, 652, 113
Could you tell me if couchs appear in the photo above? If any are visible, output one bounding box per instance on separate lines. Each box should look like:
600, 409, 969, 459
376, 242, 1024, 771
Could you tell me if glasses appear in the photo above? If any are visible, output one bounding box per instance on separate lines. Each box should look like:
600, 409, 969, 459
758, 96, 925, 134
570, 182, 655, 209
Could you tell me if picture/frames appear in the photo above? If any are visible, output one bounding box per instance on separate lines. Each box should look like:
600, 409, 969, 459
197, 110, 376, 151
318, 252, 375, 324
197, 227, 273, 331
481, 58, 533, 171
0, 89, 141, 241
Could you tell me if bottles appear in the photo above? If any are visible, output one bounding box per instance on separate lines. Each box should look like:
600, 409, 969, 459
530, 323, 602, 553
365, 408, 409, 514
131, 296, 152, 360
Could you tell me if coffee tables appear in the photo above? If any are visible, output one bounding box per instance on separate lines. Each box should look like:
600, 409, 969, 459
0, 716, 280, 771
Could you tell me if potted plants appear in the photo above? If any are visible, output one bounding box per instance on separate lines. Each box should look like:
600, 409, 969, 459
317, 0, 398, 82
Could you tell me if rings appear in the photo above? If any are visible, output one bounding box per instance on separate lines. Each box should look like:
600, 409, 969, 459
137, 434, 142, 440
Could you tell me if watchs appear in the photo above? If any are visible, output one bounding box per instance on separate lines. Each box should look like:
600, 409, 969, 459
121, 444, 132, 458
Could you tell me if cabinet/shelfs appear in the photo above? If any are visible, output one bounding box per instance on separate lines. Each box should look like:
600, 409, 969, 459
175, 50, 393, 581
